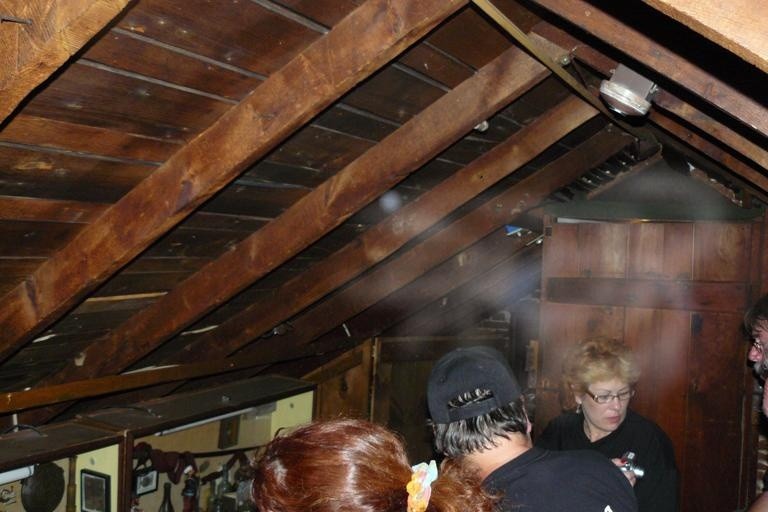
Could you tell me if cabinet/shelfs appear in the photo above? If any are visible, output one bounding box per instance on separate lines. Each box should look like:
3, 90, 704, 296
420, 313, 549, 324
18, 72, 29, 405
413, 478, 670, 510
0, 419, 125, 511
74, 377, 318, 512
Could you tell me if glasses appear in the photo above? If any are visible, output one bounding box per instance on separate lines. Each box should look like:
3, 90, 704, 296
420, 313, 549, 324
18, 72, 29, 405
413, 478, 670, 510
584, 387, 635, 405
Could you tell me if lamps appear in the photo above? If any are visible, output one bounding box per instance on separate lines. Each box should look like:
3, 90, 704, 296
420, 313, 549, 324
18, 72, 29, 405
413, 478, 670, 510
597, 62, 661, 121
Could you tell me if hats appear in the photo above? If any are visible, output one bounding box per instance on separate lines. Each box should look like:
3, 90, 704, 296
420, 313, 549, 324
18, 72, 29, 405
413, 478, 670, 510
425, 345, 522, 425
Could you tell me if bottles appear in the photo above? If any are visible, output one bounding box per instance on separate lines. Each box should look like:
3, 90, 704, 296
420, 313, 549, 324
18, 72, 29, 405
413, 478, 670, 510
182, 458, 249, 512
159, 481, 174, 511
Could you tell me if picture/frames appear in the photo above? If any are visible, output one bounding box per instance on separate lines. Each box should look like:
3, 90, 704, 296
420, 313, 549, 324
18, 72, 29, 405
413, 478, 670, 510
78, 467, 112, 511
134, 465, 160, 497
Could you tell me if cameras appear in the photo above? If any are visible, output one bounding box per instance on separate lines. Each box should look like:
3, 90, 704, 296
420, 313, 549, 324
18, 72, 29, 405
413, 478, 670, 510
619, 449, 647, 480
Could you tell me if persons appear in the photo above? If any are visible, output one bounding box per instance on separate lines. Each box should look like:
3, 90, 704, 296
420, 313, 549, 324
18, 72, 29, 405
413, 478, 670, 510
248, 410, 507, 512
423, 345, 640, 512
739, 294, 767, 512
531, 331, 684, 512
